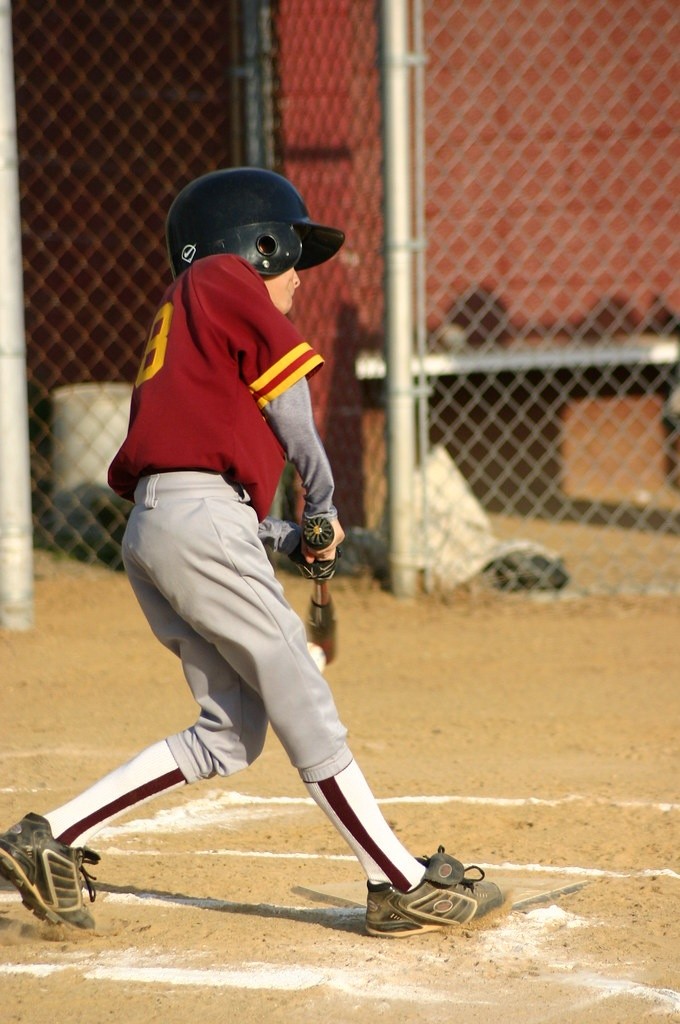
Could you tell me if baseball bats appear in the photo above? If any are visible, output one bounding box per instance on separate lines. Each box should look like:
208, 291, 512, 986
302, 516, 337, 666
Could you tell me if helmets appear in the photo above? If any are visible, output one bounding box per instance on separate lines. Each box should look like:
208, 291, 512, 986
166, 167, 346, 276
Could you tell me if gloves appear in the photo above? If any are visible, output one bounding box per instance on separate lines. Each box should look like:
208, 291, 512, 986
289, 536, 342, 581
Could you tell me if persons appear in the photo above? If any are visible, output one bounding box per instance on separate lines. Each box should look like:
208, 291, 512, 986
0, 167, 509, 939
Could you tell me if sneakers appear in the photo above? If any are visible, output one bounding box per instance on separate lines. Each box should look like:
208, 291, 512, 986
0, 812, 103, 930
366, 844, 506, 937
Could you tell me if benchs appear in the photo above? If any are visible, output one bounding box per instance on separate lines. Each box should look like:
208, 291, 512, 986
352, 333, 680, 525
